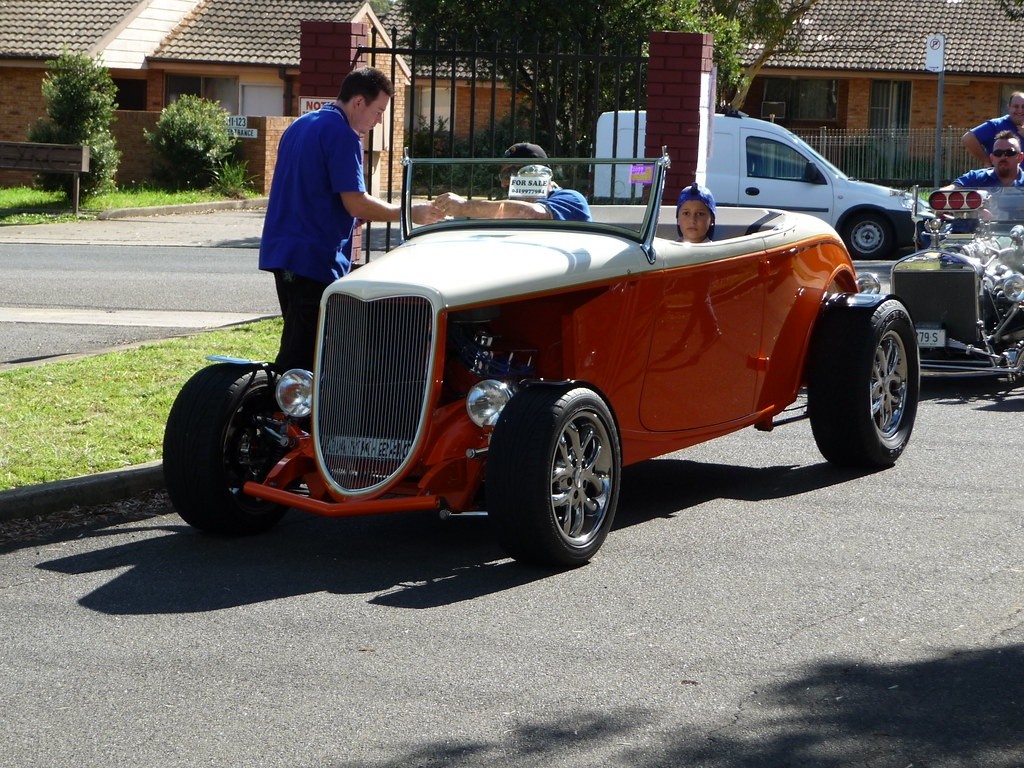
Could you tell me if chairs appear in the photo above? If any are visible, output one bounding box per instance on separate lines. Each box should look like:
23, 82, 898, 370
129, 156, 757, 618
747, 142, 763, 176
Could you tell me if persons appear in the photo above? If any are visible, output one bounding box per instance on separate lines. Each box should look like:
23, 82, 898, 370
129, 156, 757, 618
257, 67, 449, 375
938, 92, 1024, 221
676, 182, 716, 244
432, 142, 592, 222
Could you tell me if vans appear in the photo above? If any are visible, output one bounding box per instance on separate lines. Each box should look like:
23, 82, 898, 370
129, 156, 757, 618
593, 110, 936, 261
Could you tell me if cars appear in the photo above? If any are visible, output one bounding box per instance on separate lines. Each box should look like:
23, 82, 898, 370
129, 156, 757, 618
891, 186, 1024, 383
160, 147, 922, 567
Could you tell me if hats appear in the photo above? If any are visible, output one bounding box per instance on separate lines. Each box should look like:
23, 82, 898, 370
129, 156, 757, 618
676, 184, 716, 240
503, 143, 549, 170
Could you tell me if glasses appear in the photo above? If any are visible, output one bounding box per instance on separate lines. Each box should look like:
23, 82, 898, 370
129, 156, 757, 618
992, 148, 1019, 158
499, 164, 527, 182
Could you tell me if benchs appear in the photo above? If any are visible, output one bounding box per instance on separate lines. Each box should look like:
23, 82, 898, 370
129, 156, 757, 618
588, 206, 768, 243
